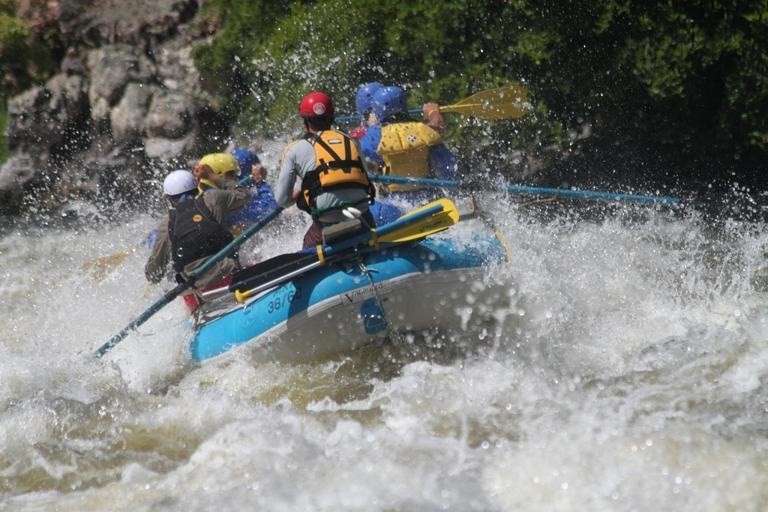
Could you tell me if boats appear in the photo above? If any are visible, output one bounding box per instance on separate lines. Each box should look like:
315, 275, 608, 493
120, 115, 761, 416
190, 226, 510, 374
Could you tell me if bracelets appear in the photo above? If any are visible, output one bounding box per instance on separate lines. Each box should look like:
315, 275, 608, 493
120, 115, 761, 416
428, 109, 438, 117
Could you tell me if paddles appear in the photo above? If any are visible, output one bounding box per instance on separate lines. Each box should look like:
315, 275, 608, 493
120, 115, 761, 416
235, 197, 459, 304
81, 174, 255, 284
335, 86, 528, 120
229, 202, 448, 293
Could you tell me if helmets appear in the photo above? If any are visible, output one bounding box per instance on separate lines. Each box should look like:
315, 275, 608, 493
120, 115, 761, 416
298, 92, 334, 118
355, 80, 386, 114
370, 85, 408, 122
231, 149, 259, 174
195, 152, 241, 196
162, 169, 197, 198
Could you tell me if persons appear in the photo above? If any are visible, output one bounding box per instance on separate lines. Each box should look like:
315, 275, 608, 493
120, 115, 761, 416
144, 165, 251, 290
274, 92, 376, 249
230, 150, 261, 180
347, 82, 387, 140
359, 87, 445, 209
198, 153, 278, 230
